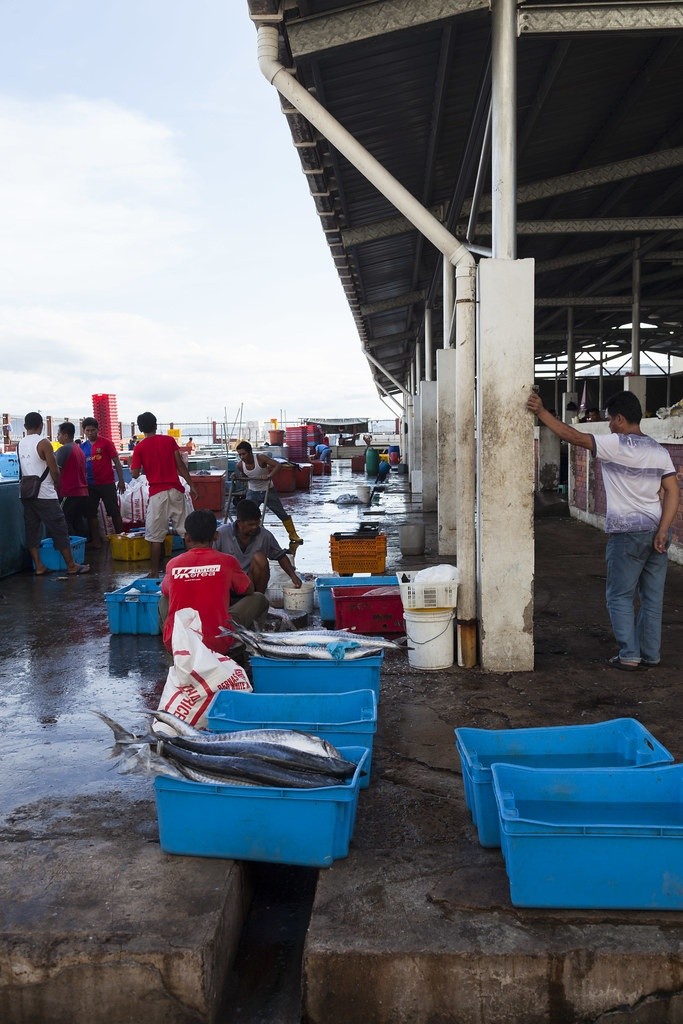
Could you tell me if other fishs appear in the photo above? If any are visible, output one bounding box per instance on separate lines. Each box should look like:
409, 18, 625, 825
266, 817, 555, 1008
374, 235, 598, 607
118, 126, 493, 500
91, 706, 367, 788
214, 617, 416, 659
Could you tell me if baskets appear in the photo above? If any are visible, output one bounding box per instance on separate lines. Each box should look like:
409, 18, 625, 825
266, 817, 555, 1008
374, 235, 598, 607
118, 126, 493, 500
396, 571, 456, 609
330, 535, 386, 573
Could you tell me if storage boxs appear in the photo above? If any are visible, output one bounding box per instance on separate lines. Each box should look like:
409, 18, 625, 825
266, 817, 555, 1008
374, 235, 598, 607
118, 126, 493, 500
329, 530, 387, 574
109, 533, 174, 561
50, 441, 64, 453
452, 717, 676, 850
315, 575, 399, 621
91, 393, 331, 511
331, 586, 406, 633
0, 450, 19, 476
352, 456, 366, 474
104, 578, 165, 636
151, 745, 370, 870
32, 535, 87, 570
249, 649, 384, 705
379, 454, 391, 464
489, 762, 683, 912
131, 527, 186, 550
206, 688, 378, 789
396, 570, 459, 610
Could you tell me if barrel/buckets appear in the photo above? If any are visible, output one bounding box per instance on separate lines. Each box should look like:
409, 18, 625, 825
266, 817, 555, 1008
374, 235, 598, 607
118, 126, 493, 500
356, 486, 371, 503
268, 430, 285, 446
282, 583, 315, 613
352, 446, 406, 476
403, 609, 456, 671
268, 554, 294, 608
398, 524, 425, 556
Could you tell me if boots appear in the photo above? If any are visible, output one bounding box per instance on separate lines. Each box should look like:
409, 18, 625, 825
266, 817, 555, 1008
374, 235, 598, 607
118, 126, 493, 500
283, 515, 302, 542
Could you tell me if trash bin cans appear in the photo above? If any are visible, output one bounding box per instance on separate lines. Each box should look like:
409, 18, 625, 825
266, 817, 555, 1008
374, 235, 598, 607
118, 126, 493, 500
398, 464, 406, 474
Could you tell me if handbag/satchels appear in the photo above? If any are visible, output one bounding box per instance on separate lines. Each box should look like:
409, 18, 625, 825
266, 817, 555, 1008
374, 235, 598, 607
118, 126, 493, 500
18, 476, 39, 500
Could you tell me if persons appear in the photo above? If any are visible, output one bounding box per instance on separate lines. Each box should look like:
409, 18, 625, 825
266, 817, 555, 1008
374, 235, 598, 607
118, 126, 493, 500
580, 408, 601, 422
212, 499, 302, 593
16, 412, 91, 574
231, 441, 303, 542
79, 417, 126, 549
526, 391, 679, 670
75, 439, 82, 445
128, 436, 140, 450
157, 509, 269, 683
129, 411, 198, 578
313, 444, 332, 464
54, 422, 90, 545
186, 438, 195, 447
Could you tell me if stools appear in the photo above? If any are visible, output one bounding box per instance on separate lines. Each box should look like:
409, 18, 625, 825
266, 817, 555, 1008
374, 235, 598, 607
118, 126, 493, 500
557, 484, 567, 494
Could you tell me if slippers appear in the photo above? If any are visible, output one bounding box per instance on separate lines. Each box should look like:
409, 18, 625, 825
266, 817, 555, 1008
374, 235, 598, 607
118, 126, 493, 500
65, 565, 90, 574
641, 657, 659, 667
606, 654, 638, 671
36, 567, 52, 576
